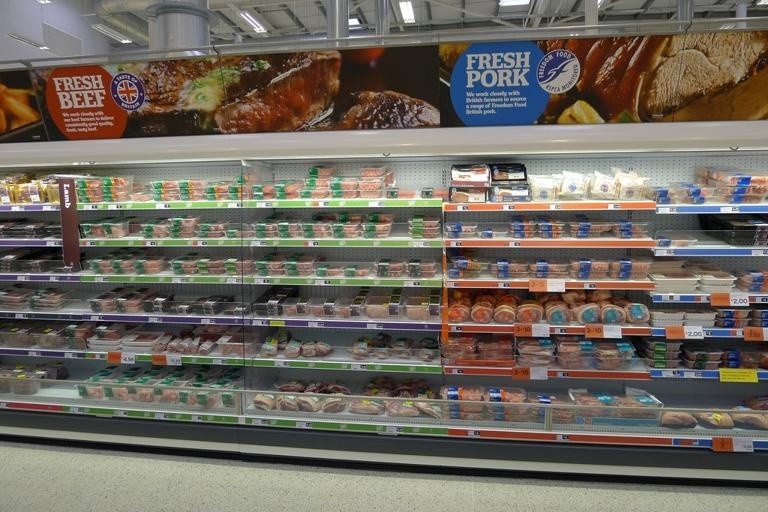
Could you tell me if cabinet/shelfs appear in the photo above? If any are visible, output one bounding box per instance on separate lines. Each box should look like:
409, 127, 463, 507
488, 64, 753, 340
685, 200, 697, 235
0, 134, 767, 487
